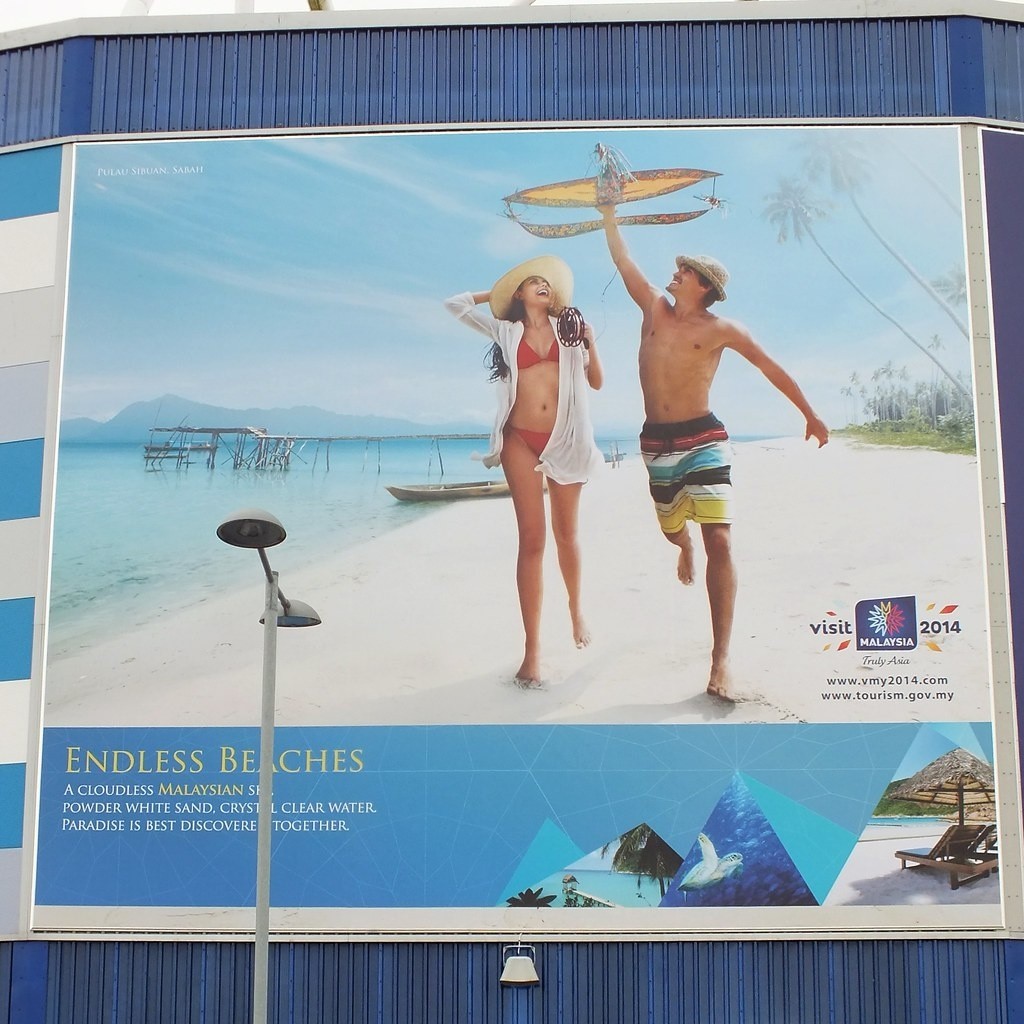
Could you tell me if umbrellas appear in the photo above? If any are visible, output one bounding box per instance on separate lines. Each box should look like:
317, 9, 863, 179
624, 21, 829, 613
881, 747, 995, 824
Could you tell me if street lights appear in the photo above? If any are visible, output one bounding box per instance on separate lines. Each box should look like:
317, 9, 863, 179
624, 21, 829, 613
212, 508, 323, 1023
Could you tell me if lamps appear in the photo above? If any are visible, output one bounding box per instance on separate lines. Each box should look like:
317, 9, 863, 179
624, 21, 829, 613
501, 934, 538, 986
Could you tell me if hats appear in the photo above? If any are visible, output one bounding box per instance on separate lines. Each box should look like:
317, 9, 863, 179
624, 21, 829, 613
676, 255, 728, 302
489, 256, 572, 320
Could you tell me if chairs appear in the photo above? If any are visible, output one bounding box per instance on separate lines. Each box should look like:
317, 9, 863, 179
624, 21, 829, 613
896, 823, 998, 890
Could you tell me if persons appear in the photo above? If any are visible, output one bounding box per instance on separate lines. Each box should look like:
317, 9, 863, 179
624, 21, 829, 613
443, 255, 603, 690
597, 202, 828, 702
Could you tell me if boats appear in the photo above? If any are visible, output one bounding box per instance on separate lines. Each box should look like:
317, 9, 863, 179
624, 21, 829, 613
385, 479, 550, 501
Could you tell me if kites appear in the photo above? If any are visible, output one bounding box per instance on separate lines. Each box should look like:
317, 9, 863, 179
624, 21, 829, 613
501, 143, 723, 239
